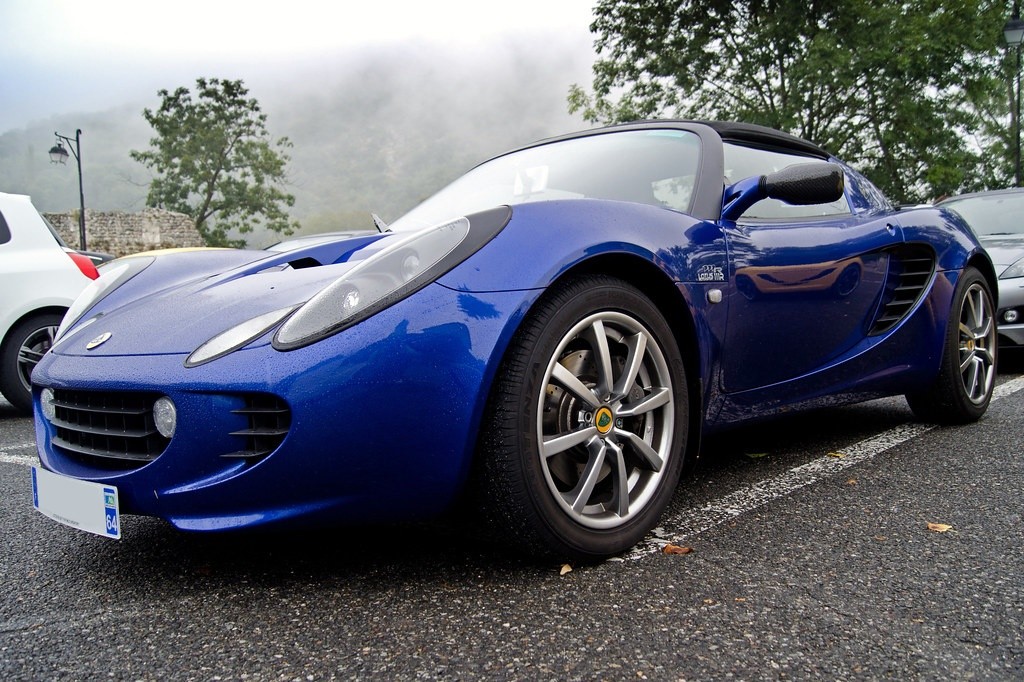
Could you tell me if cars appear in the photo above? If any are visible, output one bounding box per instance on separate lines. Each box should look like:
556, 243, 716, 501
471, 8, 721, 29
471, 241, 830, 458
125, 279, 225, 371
932, 186, 1023, 360
0, 192, 103, 413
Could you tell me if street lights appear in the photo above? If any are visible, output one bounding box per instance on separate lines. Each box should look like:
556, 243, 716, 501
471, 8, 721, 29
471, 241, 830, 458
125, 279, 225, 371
49, 128, 88, 251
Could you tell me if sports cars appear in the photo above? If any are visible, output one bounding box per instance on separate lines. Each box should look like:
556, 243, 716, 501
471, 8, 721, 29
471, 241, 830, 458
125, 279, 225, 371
23, 120, 1002, 567
